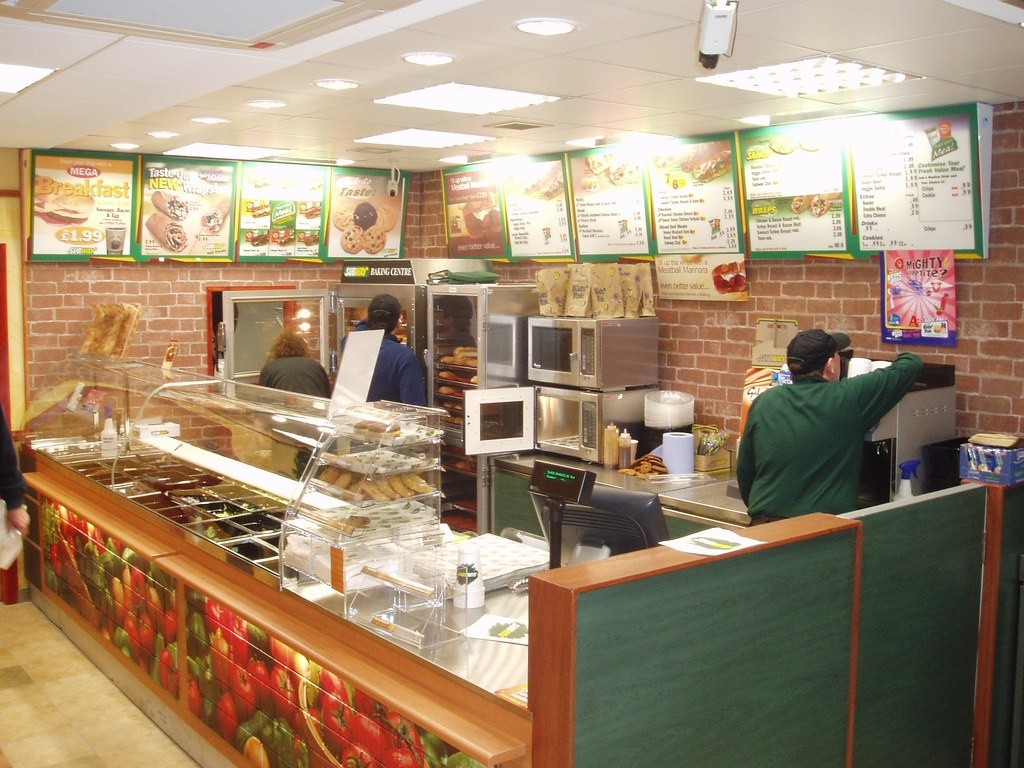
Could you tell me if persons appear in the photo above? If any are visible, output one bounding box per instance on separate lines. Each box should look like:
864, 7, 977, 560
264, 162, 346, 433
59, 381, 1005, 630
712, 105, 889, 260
0, 402, 31, 536
339, 292, 428, 407
434, 297, 477, 355
258, 333, 331, 400
737, 330, 922, 527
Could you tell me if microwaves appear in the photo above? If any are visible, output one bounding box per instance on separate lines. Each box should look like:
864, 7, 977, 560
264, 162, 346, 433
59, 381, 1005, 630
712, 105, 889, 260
488, 311, 530, 383
461, 386, 662, 465
526, 314, 659, 392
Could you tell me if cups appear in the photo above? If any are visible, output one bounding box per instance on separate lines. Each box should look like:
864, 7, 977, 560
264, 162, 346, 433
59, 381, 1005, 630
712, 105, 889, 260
453, 543, 488, 609
630, 439, 638, 466
105, 226, 127, 256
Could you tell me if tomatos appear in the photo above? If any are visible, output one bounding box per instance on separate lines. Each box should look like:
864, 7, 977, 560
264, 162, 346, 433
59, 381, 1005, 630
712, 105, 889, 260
51, 508, 123, 578
206, 597, 304, 743
124, 567, 179, 700
308, 667, 425, 768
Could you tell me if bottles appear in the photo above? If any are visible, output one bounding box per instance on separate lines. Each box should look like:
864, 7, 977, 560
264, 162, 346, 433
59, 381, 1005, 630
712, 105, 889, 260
100, 405, 119, 459
603, 420, 620, 471
618, 428, 631, 471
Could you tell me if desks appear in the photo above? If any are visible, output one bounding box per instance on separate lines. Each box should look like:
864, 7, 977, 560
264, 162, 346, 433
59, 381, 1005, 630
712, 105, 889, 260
19, 437, 573, 768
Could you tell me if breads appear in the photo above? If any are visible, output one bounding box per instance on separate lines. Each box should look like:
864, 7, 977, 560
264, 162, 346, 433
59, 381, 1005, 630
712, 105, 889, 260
316, 467, 431, 500
438, 346, 478, 425
354, 420, 401, 439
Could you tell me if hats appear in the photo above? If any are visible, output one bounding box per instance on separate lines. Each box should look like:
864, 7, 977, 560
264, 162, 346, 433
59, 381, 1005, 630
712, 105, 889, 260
787, 328, 851, 376
368, 294, 402, 316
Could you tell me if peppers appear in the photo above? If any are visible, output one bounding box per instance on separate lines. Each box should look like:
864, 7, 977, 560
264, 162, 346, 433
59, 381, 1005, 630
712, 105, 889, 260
81, 538, 124, 625
187, 590, 213, 719
238, 711, 299, 768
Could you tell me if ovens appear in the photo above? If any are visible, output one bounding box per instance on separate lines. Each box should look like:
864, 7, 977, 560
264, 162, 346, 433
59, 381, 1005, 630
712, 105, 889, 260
425, 278, 541, 465
330, 256, 497, 373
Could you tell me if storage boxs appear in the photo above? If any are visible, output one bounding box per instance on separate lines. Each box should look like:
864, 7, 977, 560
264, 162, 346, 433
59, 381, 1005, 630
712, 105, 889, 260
957, 437, 1023, 486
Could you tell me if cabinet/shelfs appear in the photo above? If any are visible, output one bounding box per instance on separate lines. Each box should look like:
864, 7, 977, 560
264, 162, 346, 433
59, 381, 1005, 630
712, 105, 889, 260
421, 278, 540, 541
16, 357, 442, 589
338, 280, 428, 361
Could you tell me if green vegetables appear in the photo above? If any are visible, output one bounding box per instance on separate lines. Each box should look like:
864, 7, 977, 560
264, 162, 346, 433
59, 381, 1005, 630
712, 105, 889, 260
42, 503, 60, 594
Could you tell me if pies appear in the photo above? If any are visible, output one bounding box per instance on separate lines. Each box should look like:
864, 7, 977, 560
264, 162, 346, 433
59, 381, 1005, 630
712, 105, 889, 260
145, 191, 230, 252
790, 194, 844, 217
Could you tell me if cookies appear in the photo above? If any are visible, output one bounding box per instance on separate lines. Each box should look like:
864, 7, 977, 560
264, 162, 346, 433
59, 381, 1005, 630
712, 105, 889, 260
333, 203, 396, 253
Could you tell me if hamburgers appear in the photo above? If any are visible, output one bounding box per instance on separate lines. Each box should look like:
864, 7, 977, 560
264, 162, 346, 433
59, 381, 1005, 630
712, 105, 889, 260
679, 142, 733, 181
711, 262, 748, 294
34, 192, 95, 225
